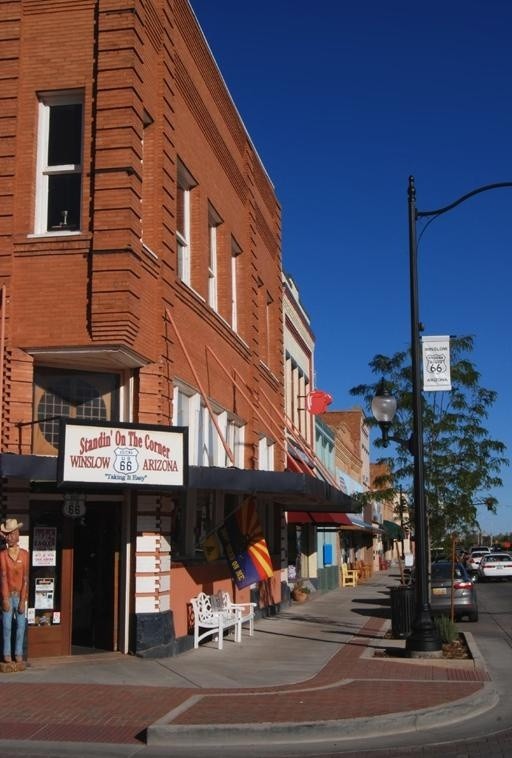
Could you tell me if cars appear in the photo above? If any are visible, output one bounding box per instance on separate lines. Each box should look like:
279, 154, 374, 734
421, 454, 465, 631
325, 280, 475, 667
429, 542, 512, 582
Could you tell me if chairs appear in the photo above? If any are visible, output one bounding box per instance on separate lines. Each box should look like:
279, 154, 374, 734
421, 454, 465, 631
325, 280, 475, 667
189, 590, 258, 650
341, 559, 372, 588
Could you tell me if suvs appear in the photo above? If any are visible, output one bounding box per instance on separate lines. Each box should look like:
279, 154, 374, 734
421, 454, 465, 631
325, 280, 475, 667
428, 557, 480, 621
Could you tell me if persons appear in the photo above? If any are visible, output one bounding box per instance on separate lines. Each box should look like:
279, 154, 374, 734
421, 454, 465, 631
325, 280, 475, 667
0, 517, 31, 674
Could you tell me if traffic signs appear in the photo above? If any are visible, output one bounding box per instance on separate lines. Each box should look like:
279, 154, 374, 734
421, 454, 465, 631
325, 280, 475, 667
422, 335, 451, 391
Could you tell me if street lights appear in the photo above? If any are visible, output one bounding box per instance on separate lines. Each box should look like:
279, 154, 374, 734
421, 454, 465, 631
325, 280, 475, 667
369, 343, 445, 659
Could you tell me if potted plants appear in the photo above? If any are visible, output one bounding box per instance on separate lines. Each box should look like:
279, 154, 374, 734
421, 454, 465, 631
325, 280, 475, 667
293, 587, 311, 600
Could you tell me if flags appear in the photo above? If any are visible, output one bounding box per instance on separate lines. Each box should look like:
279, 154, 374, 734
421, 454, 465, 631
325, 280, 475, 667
216, 499, 274, 592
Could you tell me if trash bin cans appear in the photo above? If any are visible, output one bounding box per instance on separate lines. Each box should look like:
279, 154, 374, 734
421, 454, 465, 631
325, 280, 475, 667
386, 585, 418, 640
